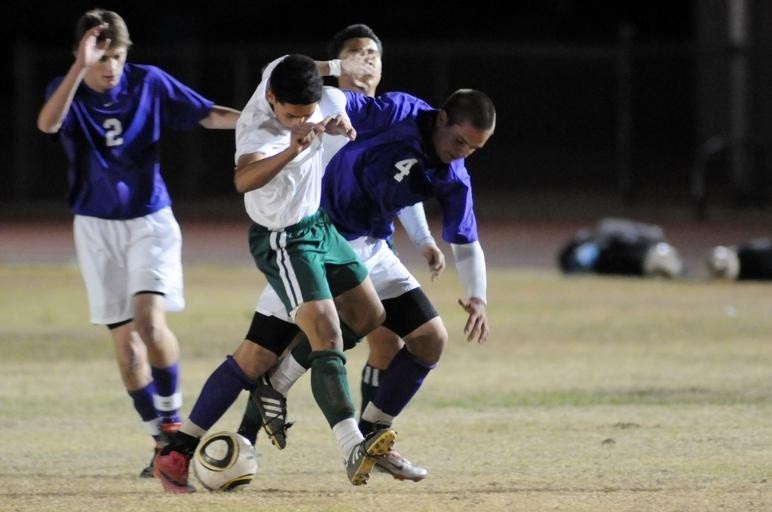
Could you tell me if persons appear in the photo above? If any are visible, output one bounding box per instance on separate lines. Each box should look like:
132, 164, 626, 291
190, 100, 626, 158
38, 8, 241, 476
234, 21, 444, 484
154, 86, 497, 498
230, 44, 399, 485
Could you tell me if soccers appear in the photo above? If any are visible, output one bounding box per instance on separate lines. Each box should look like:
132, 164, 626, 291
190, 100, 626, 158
192, 430, 256, 492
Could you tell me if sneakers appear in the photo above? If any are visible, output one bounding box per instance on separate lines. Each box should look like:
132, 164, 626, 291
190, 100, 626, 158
373, 448, 428, 482
345, 426, 398, 487
139, 421, 183, 479
151, 448, 197, 494
249, 372, 294, 451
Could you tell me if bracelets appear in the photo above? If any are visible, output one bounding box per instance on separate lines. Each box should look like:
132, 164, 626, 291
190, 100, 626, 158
328, 58, 342, 77
